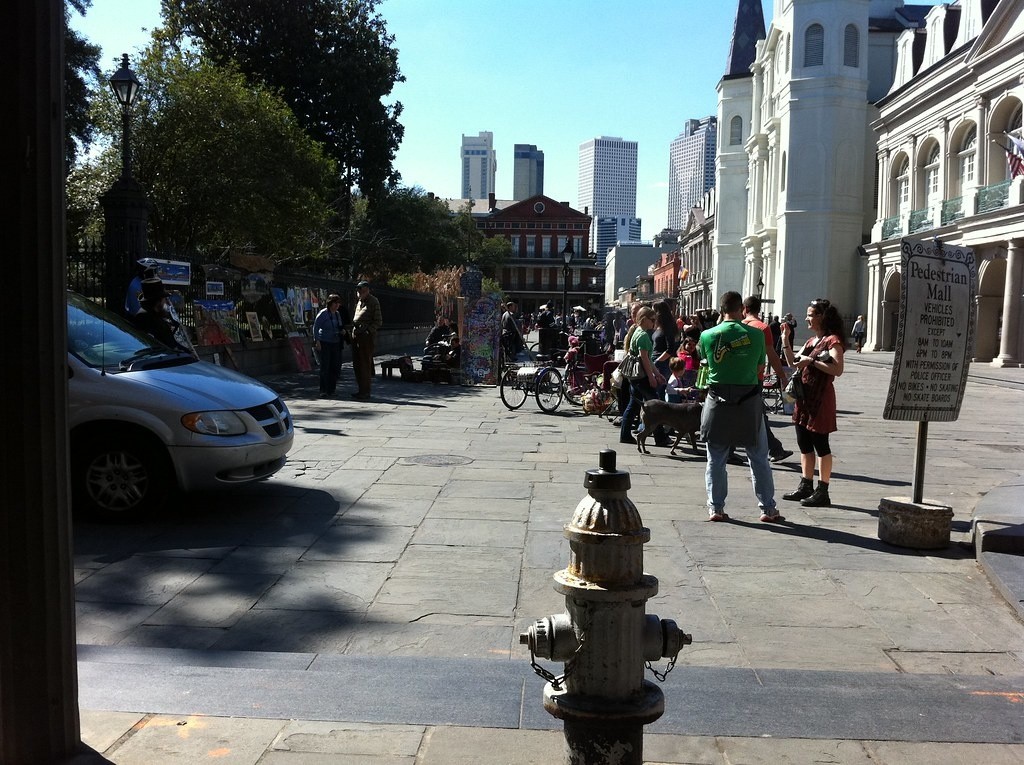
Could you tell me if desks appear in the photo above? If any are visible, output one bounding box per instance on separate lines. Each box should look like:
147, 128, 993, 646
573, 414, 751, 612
381, 360, 412, 378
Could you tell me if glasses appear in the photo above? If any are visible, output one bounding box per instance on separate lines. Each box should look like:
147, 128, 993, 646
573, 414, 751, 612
643, 314, 655, 324
809, 300, 820, 308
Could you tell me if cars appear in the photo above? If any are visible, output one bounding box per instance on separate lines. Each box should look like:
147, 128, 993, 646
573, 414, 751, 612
68, 308, 298, 514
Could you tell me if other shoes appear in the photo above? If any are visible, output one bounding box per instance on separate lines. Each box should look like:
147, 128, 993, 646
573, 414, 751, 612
619, 436, 638, 444
331, 391, 343, 399
771, 450, 794, 463
353, 393, 370, 401
655, 439, 673, 446
318, 392, 329, 399
727, 454, 745, 465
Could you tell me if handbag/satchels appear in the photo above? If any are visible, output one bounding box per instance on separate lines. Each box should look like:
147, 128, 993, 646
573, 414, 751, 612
617, 351, 646, 381
785, 365, 816, 402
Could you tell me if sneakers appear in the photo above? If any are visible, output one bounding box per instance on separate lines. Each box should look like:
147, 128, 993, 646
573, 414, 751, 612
708, 507, 725, 520
760, 509, 781, 521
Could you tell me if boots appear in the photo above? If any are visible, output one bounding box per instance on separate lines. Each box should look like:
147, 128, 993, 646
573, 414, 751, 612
781, 477, 815, 501
800, 480, 832, 507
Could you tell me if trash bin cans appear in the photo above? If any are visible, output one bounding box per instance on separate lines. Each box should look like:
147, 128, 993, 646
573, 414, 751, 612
539, 328, 557, 355
582, 330, 600, 355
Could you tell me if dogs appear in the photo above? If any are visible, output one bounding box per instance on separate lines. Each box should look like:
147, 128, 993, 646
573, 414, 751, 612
630, 393, 706, 455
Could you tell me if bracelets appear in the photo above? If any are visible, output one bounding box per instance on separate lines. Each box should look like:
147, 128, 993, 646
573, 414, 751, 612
782, 345, 790, 348
811, 357, 815, 367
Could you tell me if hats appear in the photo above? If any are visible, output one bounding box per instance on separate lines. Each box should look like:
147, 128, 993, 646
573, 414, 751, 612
137, 278, 171, 301
355, 281, 368, 289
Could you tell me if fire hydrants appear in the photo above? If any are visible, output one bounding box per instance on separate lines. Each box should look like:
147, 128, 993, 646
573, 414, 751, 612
515, 442, 693, 763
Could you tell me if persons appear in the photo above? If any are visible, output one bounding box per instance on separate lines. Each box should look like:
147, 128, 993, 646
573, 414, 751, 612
851, 314, 865, 353
350, 281, 382, 399
700, 292, 780, 521
501, 301, 525, 361
782, 298, 846, 506
313, 294, 347, 397
525, 312, 537, 334
125, 257, 190, 354
539, 299, 615, 358
726, 296, 794, 463
767, 312, 797, 360
665, 316, 719, 444
425, 315, 460, 383
565, 335, 580, 393
613, 301, 677, 446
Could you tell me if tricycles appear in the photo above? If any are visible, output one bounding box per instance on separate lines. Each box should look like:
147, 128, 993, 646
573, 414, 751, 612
499, 348, 594, 413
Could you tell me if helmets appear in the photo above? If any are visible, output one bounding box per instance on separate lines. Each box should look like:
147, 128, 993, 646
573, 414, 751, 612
138, 258, 159, 271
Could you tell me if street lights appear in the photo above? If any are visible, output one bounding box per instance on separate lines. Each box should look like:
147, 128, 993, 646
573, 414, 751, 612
559, 239, 575, 346
95, 57, 150, 314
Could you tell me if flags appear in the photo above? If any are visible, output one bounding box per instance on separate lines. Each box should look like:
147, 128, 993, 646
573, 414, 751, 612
1006, 150, 1024, 179
1007, 134, 1024, 158
678, 266, 689, 281
